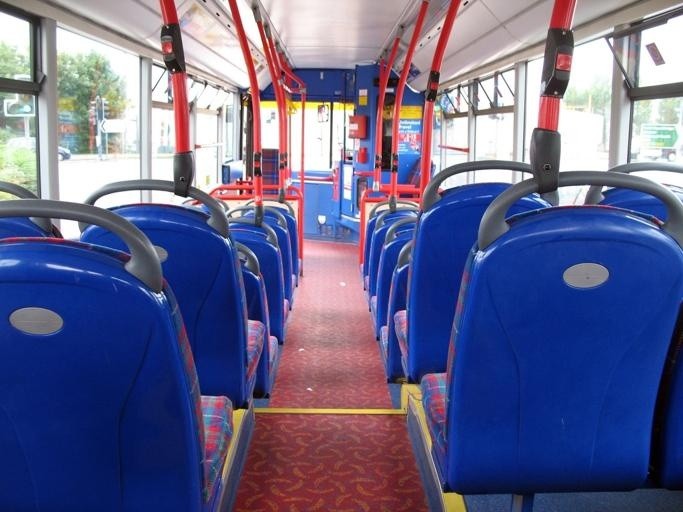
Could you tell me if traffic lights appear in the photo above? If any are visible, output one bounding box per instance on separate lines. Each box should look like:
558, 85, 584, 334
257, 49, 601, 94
24, 95, 33, 113
104, 101, 109, 119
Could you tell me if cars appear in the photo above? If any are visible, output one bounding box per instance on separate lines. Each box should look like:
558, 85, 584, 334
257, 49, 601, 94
8, 137, 71, 161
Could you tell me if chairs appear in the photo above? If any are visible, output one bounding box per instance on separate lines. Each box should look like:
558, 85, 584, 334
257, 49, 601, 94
2, 161, 308, 511
351, 162, 683, 510
